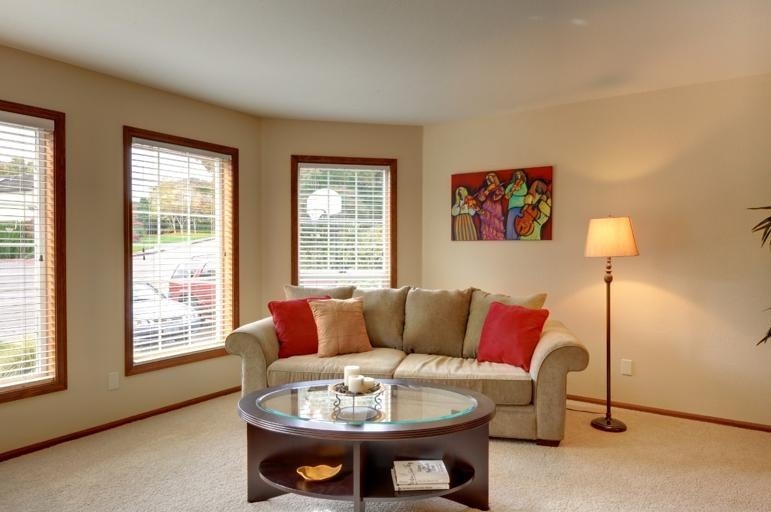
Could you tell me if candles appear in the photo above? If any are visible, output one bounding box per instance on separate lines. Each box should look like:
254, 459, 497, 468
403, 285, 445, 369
343, 365, 376, 394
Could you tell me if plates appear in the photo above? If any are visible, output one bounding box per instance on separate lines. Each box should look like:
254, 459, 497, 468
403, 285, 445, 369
331, 406, 386, 423
327, 381, 385, 397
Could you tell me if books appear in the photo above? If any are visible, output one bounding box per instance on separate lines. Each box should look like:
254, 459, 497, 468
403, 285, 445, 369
388, 457, 453, 493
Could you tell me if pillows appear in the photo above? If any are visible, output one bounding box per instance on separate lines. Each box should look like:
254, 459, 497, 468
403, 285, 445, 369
268, 283, 551, 374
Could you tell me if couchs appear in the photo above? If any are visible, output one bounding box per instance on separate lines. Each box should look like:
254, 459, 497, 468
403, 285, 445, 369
224, 314, 591, 448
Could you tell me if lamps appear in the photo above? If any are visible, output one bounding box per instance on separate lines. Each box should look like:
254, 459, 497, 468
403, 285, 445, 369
583, 210, 642, 434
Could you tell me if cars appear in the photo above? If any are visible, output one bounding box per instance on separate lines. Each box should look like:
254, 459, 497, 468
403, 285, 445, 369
169, 255, 216, 321
131, 280, 198, 361
